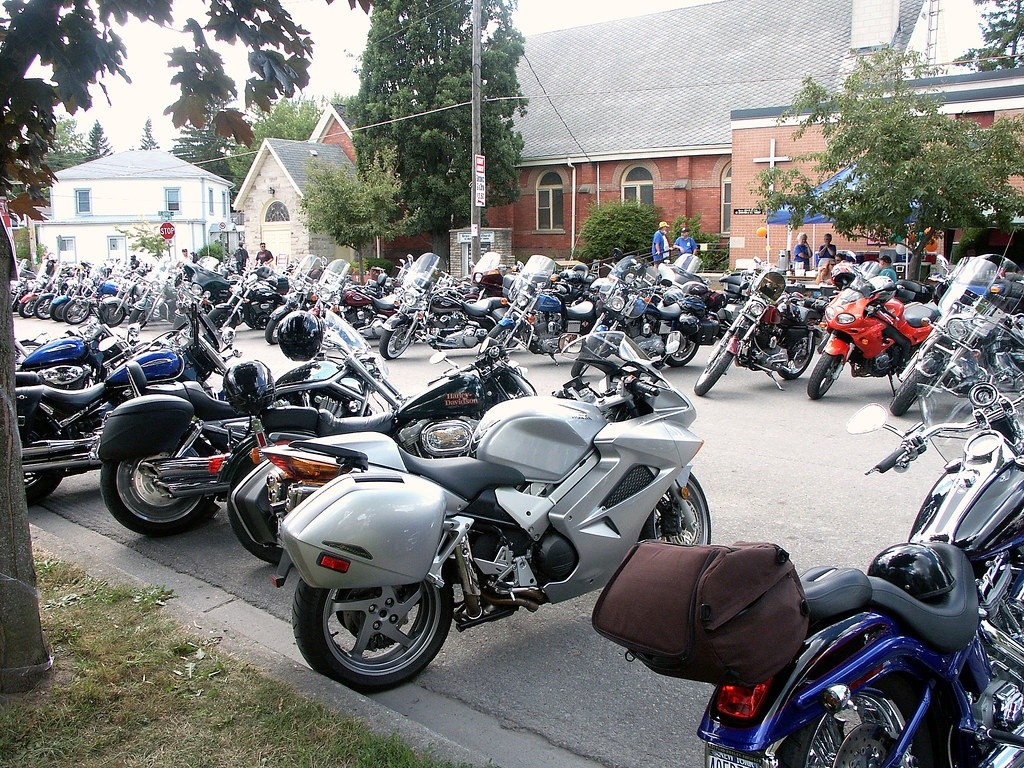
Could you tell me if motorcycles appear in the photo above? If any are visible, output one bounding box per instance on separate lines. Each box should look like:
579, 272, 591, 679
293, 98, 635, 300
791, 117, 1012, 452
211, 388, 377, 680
259, 330, 712, 693
4, 253, 1024, 417
96, 310, 405, 536
216, 335, 538, 565
695, 315, 1024, 768
14, 293, 243, 508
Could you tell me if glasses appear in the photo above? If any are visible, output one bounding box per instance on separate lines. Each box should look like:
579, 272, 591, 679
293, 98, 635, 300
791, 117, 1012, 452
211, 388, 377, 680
262, 246, 265, 247
184, 251, 187, 252
829, 264, 836, 265
837, 260, 842, 261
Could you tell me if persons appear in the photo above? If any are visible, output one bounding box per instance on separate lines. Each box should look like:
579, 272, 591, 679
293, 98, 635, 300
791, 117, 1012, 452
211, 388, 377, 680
652, 219, 674, 270
877, 255, 897, 282
176, 249, 193, 267
130, 255, 141, 269
232, 241, 252, 278
793, 232, 813, 272
254, 243, 274, 268
37, 249, 58, 278
816, 233, 845, 285
673, 227, 699, 255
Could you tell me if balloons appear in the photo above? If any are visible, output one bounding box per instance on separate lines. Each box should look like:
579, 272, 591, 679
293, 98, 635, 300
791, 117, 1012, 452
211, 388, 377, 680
756, 226, 767, 238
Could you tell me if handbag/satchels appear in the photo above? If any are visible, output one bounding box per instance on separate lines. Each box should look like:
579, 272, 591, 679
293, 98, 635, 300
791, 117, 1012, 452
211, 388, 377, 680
590, 540, 811, 692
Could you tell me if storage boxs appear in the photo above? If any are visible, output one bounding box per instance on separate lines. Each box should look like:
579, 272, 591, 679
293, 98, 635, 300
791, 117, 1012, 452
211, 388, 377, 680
97, 395, 193, 462
278, 474, 444, 589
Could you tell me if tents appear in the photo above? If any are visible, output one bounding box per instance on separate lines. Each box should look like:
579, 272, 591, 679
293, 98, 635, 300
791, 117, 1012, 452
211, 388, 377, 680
766, 153, 1024, 270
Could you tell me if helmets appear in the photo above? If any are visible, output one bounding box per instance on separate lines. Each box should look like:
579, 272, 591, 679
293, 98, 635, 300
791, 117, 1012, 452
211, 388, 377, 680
223, 360, 275, 414
378, 274, 388, 286
277, 310, 323, 361
867, 541, 958, 600
932, 282, 949, 306
830, 264, 856, 291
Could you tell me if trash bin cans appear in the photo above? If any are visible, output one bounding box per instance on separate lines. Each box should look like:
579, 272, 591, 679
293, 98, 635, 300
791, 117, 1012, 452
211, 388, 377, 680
816, 252, 822, 267
919, 262, 931, 281
779, 250, 790, 272
898, 250, 936, 264
893, 261, 907, 280
855, 252, 879, 268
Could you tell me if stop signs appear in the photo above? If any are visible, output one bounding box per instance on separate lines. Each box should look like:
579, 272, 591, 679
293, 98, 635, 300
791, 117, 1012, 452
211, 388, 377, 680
160, 223, 175, 239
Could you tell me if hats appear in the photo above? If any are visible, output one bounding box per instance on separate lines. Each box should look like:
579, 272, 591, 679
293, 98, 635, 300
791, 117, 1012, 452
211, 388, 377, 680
877, 255, 891, 263
680, 227, 689, 232
658, 222, 669, 230
